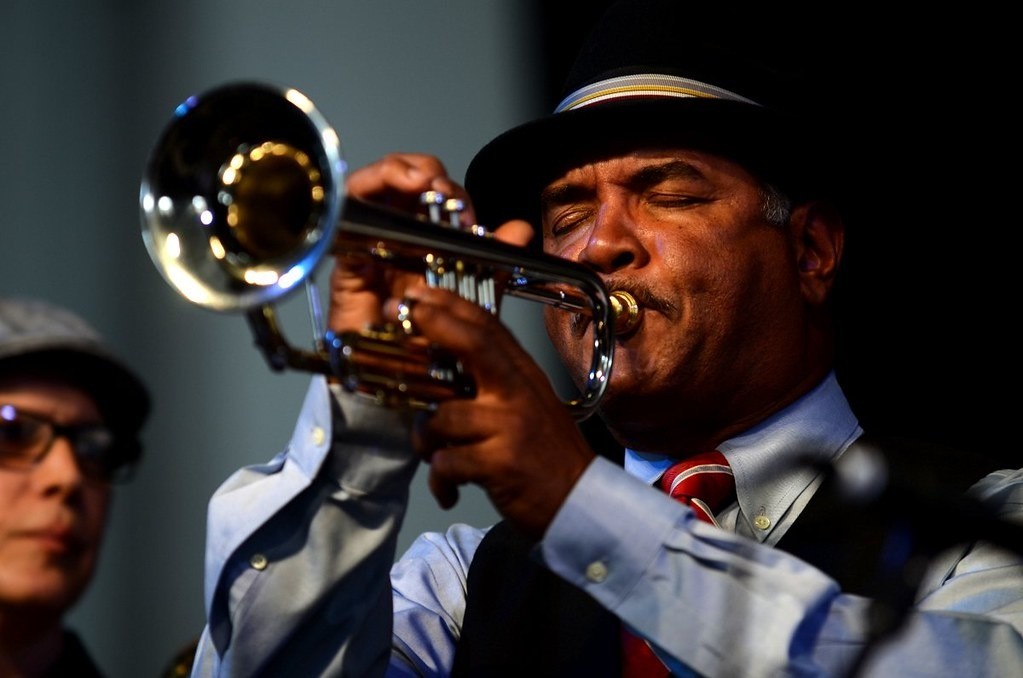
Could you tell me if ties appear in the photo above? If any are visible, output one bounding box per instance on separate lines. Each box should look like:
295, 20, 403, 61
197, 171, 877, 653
618, 447, 736, 678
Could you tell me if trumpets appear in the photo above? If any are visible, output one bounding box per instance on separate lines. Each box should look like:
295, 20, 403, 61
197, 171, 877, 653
137, 77, 643, 416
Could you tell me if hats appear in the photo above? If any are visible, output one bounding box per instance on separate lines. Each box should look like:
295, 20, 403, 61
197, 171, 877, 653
0, 295, 154, 442
461, 38, 846, 207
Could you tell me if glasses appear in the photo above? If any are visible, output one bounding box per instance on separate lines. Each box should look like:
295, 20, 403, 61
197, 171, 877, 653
0, 406, 144, 485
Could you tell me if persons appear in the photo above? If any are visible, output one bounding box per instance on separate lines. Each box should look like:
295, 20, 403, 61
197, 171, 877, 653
0, 293, 156, 678
188, 28, 1023, 678
